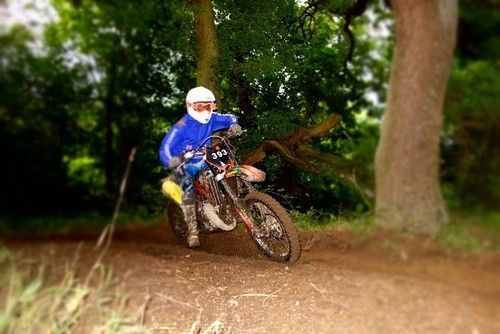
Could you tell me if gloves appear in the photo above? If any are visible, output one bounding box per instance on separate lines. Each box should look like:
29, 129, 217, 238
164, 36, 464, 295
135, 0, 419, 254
169, 157, 181, 169
228, 124, 242, 137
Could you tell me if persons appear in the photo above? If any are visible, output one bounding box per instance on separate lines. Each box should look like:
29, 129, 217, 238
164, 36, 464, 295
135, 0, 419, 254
158, 86, 243, 248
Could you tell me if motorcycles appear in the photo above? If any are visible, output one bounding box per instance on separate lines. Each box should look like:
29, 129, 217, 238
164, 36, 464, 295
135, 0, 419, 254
160, 130, 302, 265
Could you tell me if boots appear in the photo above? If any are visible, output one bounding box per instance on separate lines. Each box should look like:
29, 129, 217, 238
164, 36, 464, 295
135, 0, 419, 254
177, 203, 200, 248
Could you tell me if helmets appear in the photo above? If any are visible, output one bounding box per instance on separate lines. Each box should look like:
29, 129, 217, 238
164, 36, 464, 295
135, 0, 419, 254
185, 85, 216, 124
